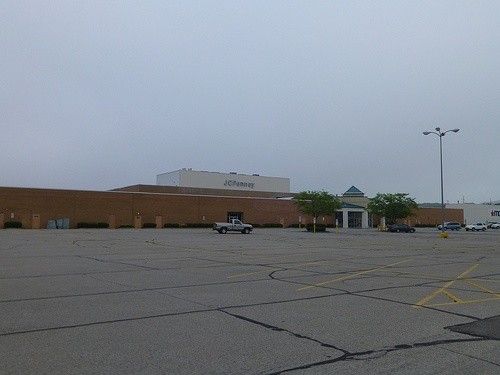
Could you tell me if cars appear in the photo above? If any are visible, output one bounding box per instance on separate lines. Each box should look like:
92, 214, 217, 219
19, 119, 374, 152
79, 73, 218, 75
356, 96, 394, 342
389, 223, 416, 233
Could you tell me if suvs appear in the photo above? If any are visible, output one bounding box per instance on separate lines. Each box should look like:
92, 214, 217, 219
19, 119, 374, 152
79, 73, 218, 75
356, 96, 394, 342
438, 222, 461, 231
465, 222, 486, 231
487, 222, 497, 227
491, 222, 500, 229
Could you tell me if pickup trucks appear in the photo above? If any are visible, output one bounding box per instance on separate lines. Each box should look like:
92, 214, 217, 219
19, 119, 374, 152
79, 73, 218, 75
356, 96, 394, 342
213, 219, 253, 234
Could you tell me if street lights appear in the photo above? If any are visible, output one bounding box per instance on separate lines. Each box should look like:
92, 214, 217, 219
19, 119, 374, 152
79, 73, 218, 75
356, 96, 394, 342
423, 127, 460, 238
